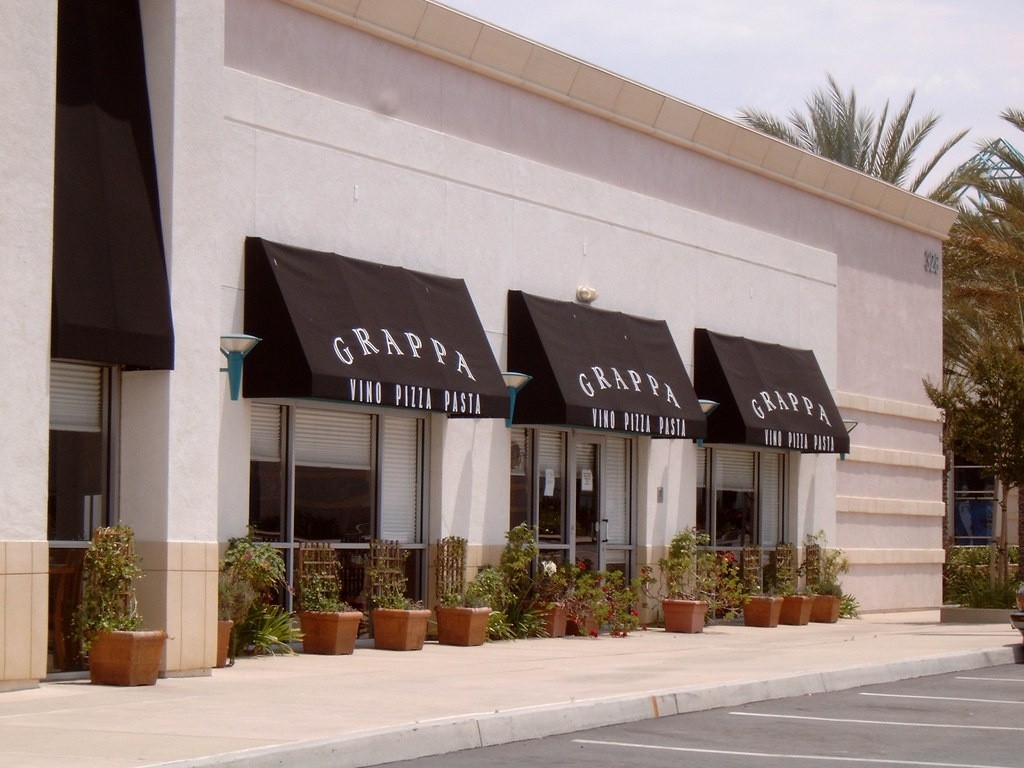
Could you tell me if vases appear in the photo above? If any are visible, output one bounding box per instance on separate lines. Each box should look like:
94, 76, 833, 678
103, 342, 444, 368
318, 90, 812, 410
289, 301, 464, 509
218, 617, 234, 667
542, 602, 570, 638
570, 599, 601, 636
662, 597, 710, 634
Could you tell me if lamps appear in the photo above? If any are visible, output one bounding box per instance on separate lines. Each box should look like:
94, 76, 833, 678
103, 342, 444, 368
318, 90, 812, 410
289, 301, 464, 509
842, 419, 861, 462
501, 372, 533, 425
576, 285, 599, 301
696, 399, 718, 449
218, 330, 264, 398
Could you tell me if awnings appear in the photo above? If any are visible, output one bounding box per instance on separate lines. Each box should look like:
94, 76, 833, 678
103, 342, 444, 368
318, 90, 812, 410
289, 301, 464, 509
693, 328, 850, 453
242, 236, 511, 418
507, 290, 707, 439
49, 0, 174, 371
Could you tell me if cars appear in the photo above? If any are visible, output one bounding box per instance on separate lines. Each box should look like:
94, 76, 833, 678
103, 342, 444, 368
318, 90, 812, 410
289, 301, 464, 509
1010, 584, 1024, 637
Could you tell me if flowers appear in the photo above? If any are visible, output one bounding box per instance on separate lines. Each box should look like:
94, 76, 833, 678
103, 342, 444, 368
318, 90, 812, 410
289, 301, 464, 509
541, 544, 738, 640
218, 536, 289, 617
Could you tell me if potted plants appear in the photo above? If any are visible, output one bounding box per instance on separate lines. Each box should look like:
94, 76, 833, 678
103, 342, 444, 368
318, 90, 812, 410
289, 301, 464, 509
777, 546, 815, 625
741, 544, 785, 628
83, 522, 167, 685
807, 531, 855, 623
369, 545, 432, 651
435, 566, 501, 646
300, 550, 364, 655
